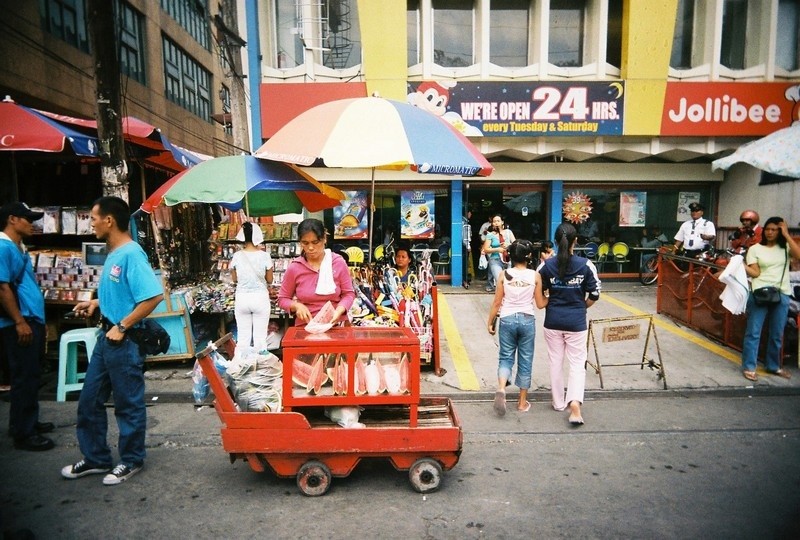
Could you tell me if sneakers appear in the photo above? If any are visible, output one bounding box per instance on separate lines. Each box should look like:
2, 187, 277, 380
61, 459, 113, 478
102, 462, 143, 485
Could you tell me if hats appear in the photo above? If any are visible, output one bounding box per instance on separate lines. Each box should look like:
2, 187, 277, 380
688, 202, 704, 211
5, 201, 44, 220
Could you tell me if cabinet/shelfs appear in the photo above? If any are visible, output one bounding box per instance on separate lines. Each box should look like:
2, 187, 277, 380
281, 324, 421, 427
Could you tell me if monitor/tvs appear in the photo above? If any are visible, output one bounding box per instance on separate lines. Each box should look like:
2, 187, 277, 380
82, 242, 107, 268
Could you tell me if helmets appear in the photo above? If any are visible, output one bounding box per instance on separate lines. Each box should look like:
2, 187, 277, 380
740, 210, 759, 224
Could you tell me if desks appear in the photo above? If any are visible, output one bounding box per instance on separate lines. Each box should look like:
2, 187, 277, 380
630, 246, 659, 268
409, 248, 439, 253
573, 247, 593, 251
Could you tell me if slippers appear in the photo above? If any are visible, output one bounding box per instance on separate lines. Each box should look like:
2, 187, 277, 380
569, 411, 584, 424
766, 368, 792, 378
518, 400, 531, 413
743, 368, 758, 381
493, 390, 506, 416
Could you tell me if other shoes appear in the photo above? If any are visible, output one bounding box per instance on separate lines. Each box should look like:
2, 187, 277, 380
12, 434, 54, 451
485, 286, 495, 292
36, 422, 55, 432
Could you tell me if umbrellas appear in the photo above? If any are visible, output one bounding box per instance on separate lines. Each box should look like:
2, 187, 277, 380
0, 95, 101, 201
129, 152, 347, 221
352, 253, 436, 336
252, 93, 495, 263
711, 126, 800, 178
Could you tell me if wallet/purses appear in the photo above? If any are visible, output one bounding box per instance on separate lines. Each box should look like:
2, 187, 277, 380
492, 316, 498, 347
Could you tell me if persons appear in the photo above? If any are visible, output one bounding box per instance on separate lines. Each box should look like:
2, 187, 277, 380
0, 200, 55, 452
62, 196, 163, 486
230, 202, 799, 426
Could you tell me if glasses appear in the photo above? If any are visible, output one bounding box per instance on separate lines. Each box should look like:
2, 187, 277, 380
690, 208, 702, 212
20, 217, 33, 223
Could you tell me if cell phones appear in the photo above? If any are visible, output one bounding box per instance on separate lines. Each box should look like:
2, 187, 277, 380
65, 309, 87, 318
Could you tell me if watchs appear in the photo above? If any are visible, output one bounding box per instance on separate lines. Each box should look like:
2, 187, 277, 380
116, 320, 127, 333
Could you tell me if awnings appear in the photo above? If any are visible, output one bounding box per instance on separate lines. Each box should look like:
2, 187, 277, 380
30, 106, 206, 175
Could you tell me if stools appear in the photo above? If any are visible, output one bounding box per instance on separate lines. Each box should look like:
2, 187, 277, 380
56, 327, 104, 402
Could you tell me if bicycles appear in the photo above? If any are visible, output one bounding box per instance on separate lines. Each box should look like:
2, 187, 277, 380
638, 236, 739, 320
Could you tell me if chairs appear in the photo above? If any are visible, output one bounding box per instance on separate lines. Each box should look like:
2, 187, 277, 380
593, 242, 609, 273
583, 242, 598, 260
433, 248, 452, 275
611, 242, 630, 274
436, 243, 450, 275
411, 244, 430, 263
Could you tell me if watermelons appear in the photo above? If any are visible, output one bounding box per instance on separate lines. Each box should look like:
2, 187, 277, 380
355, 353, 369, 396
304, 301, 335, 333
375, 357, 389, 394
398, 353, 410, 394
292, 353, 348, 396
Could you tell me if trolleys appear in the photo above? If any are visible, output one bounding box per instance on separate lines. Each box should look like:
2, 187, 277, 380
199, 329, 463, 497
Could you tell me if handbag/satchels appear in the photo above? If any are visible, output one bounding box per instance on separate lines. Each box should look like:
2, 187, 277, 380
225, 345, 283, 413
142, 318, 171, 355
0, 282, 21, 318
191, 341, 230, 410
478, 254, 488, 270
323, 402, 368, 429
753, 286, 782, 305
